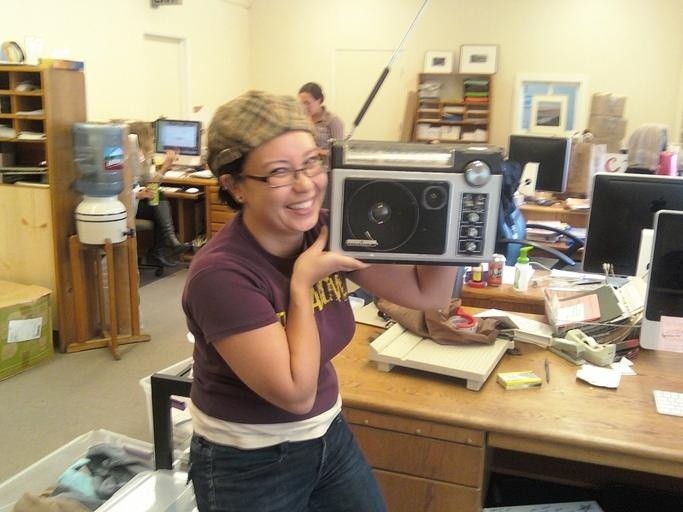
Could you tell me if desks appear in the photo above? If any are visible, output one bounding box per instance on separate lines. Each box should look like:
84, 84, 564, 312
518, 192, 589, 262
462, 267, 578, 316
330, 305, 683, 512
156, 165, 236, 243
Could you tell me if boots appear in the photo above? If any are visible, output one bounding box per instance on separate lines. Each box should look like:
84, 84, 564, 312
148, 249, 178, 269
155, 201, 191, 254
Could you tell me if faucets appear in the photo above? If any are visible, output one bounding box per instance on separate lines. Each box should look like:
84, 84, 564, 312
123, 227, 136, 240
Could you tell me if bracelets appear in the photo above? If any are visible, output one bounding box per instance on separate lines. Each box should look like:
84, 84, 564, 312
161, 165, 171, 170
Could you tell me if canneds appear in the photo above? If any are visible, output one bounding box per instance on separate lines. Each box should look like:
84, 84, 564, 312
488, 254, 504, 287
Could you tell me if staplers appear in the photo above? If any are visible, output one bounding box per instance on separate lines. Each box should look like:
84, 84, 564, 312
548, 336, 586, 367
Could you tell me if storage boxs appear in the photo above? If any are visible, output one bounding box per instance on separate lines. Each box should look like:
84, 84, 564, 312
140, 355, 194, 453
0, 280, 54, 383
588, 93, 627, 154
0, 428, 199, 512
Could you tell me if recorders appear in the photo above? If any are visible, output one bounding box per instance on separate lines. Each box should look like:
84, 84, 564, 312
330, 141, 503, 266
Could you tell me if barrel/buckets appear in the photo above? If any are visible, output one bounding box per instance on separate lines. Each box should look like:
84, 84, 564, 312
71, 121, 126, 198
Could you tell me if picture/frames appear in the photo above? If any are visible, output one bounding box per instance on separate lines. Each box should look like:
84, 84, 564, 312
458, 45, 498, 74
423, 48, 454, 74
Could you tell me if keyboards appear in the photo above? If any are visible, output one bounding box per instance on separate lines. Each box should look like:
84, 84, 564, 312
653, 390, 683, 416
159, 186, 182, 193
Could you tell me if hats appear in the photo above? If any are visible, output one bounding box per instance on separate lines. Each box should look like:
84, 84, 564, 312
207, 88, 321, 178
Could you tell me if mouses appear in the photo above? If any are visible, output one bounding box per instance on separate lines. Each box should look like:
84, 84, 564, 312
185, 188, 200, 193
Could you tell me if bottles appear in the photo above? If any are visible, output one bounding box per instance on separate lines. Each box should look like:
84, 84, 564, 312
463, 263, 490, 287
657, 150, 678, 175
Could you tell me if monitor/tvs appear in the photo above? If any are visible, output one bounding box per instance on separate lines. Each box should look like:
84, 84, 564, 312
582, 172, 683, 281
640, 209, 683, 352
156, 120, 201, 161
510, 136, 572, 197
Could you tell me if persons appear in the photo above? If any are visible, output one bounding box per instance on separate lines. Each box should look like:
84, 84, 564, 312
177, 88, 455, 511
130, 122, 191, 268
298, 83, 344, 172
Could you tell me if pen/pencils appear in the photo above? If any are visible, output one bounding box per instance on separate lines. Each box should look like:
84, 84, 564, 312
544, 357, 551, 384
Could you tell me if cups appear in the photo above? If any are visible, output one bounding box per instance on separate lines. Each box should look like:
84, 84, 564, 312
148, 183, 159, 206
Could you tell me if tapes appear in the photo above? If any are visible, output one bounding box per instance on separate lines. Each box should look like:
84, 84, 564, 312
582, 336, 610, 347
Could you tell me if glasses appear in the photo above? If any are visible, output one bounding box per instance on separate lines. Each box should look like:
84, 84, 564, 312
235, 158, 325, 189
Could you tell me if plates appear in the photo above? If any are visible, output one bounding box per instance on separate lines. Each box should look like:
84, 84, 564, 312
1, 40, 26, 64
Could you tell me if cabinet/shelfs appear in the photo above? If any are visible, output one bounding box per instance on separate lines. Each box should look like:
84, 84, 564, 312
414, 73, 492, 143
0, 64, 87, 332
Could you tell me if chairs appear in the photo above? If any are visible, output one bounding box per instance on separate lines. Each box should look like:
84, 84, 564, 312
150, 373, 195, 468
134, 217, 164, 277
